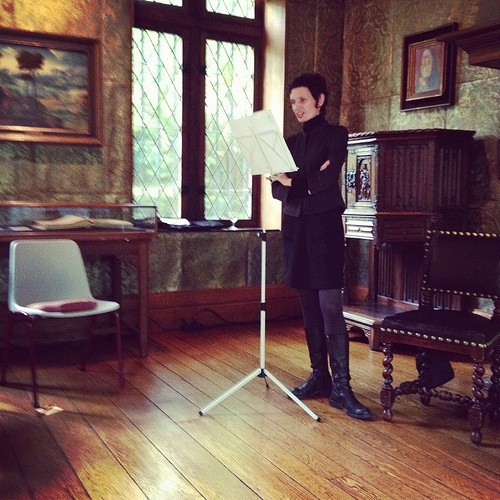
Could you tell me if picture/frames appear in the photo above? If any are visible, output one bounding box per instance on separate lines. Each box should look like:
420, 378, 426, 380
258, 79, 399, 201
0, 27, 105, 146
399, 22, 460, 113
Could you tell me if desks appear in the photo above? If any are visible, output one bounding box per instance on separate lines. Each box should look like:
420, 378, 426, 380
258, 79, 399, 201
339, 217, 475, 351
0, 201, 159, 360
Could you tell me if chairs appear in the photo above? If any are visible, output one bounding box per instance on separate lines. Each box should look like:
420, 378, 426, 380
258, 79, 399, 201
0, 239, 123, 410
379, 215, 500, 445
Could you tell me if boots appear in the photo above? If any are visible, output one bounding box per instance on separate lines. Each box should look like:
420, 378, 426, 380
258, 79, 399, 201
325, 334, 373, 421
287, 330, 333, 400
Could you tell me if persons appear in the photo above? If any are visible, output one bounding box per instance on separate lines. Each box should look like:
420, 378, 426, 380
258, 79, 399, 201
270, 74, 372, 419
